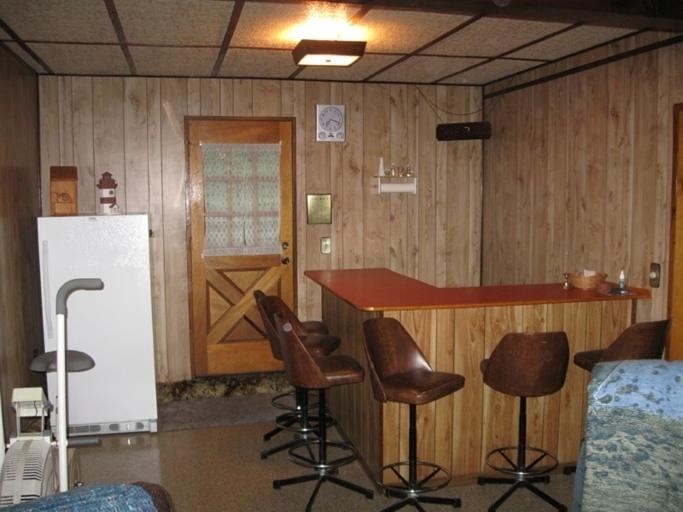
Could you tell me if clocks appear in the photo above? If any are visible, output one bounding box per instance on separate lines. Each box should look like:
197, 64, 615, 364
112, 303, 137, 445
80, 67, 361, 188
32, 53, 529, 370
315, 103, 346, 143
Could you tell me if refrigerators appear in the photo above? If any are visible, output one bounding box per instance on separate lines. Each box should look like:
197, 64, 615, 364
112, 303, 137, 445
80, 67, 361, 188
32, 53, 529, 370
37, 215, 157, 436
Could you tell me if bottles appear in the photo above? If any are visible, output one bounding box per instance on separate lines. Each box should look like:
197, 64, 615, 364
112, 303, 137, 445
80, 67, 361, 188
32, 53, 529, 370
378, 157, 411, 178
619, 270, 624, 290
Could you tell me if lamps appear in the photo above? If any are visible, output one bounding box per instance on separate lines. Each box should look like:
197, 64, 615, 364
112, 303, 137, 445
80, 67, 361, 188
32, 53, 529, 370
289, 39, 366, 69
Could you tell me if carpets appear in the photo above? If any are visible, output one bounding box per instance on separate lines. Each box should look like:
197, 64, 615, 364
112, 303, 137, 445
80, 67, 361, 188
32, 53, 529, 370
157, 393, 320, 433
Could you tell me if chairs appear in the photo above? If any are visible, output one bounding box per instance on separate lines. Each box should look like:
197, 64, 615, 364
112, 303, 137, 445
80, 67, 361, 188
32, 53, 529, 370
363, 317, 465, 511
272, 313, 374, 511
562, 319, 672, 477
476, 329, 570, 512
255, 292, 342, 461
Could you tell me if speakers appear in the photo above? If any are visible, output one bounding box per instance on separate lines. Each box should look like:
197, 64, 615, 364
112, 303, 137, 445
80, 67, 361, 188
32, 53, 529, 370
436, 121, 490, 140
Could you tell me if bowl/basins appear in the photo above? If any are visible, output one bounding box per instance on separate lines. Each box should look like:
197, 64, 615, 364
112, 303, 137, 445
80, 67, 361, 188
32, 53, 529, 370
565, 272, 608, 289
